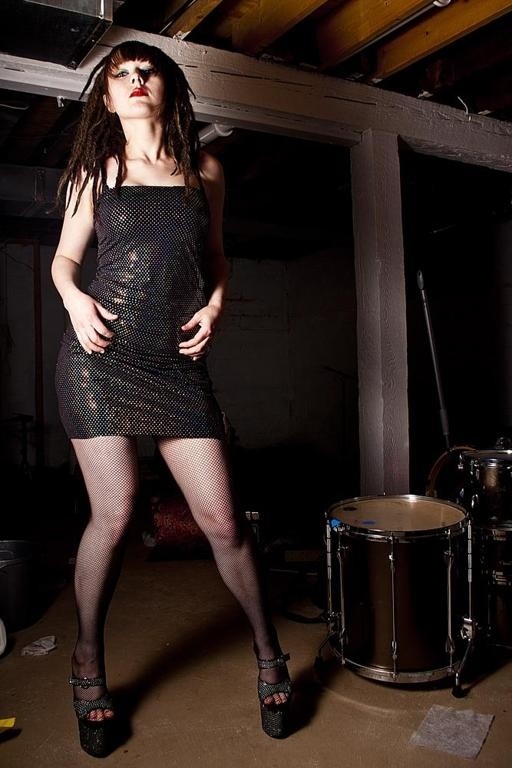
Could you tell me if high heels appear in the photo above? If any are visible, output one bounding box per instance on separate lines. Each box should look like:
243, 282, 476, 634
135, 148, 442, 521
69, 672, 114, 756
257, 653, 293, 737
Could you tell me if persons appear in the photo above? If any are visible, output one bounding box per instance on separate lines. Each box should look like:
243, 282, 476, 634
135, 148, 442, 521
49, 41, 299, 759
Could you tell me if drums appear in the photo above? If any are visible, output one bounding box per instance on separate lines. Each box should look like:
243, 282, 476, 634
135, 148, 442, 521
322, 493, 471, 683
458, 449, 511, 527
471, 527, 511, 589
425, 437, 512, 650
